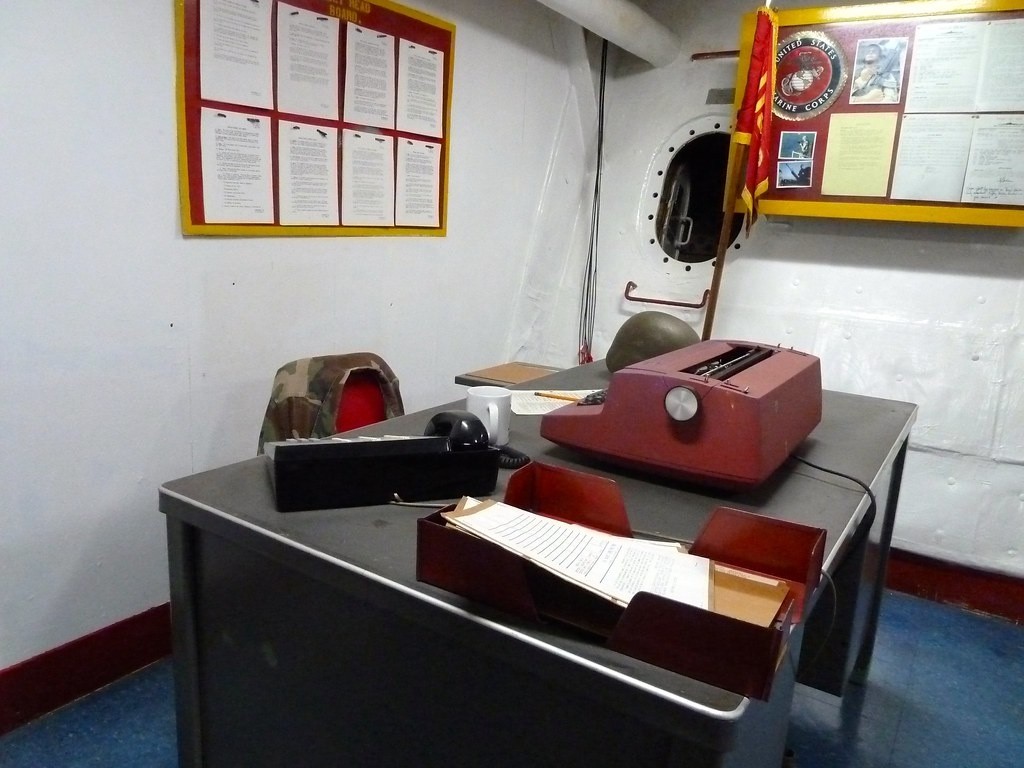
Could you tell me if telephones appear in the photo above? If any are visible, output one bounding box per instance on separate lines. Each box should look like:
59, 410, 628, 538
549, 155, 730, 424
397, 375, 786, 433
262, 406, 503, 514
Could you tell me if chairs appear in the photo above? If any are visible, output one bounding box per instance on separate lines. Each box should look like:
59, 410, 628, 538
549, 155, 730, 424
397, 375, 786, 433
335, 369, 386, 433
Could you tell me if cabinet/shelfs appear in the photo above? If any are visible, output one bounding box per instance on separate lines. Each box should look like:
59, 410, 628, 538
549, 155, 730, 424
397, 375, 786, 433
158, 357, 919, 768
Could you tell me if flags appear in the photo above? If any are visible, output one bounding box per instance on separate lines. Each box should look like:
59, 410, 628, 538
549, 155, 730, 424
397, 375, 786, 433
732, 8, 778, 237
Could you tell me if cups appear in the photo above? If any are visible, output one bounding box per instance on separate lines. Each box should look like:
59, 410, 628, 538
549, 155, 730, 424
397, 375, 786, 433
466, 385, 514, 449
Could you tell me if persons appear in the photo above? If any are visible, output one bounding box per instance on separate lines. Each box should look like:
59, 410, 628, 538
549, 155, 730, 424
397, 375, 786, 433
799, 136, 809, 156
853, 43, 896, 102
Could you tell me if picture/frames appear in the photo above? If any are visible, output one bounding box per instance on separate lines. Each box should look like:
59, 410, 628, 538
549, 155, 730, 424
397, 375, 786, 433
723, 0, 1024, 228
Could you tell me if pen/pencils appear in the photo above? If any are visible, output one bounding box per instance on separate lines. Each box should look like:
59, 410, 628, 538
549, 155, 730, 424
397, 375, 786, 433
534, 391, 582, 402
